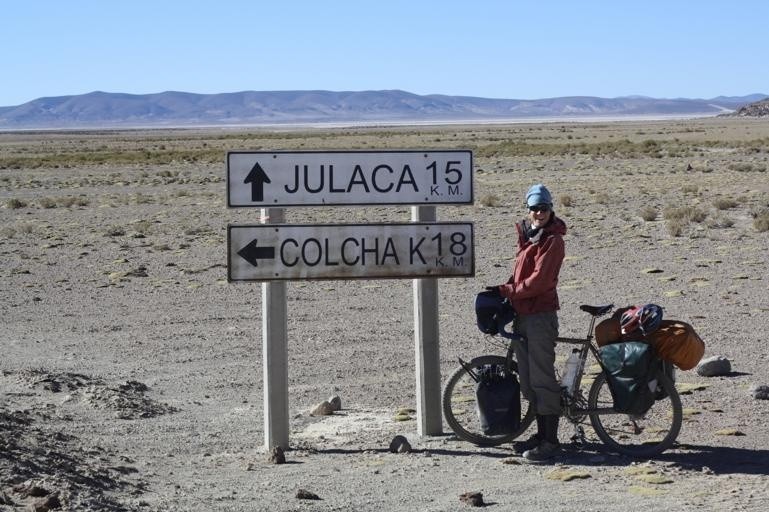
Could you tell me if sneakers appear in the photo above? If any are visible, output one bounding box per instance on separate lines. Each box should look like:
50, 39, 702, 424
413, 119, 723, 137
513, 434, 562, 461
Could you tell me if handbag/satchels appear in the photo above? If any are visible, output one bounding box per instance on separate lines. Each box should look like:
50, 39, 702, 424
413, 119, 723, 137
600, 342, 675, 414
475, 373, 520, 435
475, 292, 515, 334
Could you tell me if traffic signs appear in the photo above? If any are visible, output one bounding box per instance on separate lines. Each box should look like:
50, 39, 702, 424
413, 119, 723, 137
225, 149, 474, 209
226, 220, 475, 284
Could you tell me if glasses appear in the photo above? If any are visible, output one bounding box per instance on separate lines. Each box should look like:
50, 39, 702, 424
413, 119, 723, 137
530, 206, 550, 212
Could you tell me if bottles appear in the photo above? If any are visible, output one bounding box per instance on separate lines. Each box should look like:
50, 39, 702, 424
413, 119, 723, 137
560, 348, 579, 394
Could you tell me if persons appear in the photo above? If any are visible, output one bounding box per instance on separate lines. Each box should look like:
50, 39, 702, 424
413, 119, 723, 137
485, 183, 573, 461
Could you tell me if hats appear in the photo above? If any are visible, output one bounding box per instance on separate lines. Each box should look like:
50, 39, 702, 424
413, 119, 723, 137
526, 184, 552, 207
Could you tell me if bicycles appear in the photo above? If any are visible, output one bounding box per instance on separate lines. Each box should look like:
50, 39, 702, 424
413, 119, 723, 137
444, 286, 681, 458
